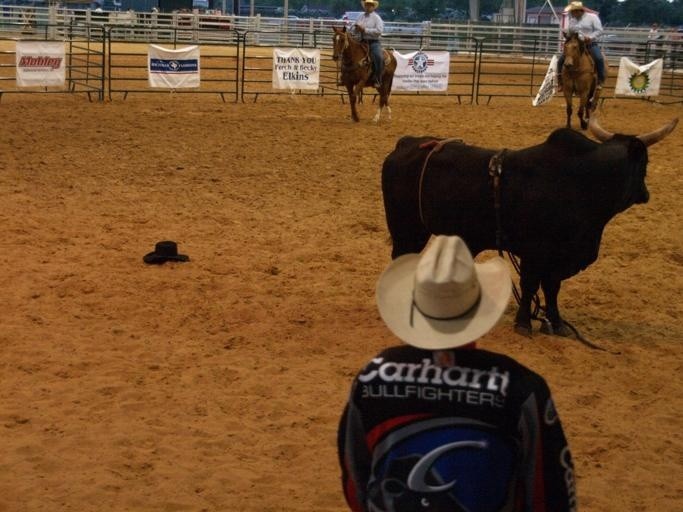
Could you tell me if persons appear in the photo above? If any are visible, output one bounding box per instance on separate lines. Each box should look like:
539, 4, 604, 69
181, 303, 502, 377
353, 0, 385, 88
648, 23, 660, 52
556, 1, 604, 92
337, 233, 577, 512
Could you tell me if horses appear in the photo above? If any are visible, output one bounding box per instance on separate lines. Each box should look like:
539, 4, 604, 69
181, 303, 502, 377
331, 24, 397, 123
558, 28, 608, 131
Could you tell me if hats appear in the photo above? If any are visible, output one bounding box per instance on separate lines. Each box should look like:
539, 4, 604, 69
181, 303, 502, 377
565, 2, 583, 11
143, 241, 188, 263
376, 234, 512, 350
361, 0, 379, 10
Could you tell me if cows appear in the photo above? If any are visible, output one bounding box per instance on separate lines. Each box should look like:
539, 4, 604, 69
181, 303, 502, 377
382, 113, 681, 337
